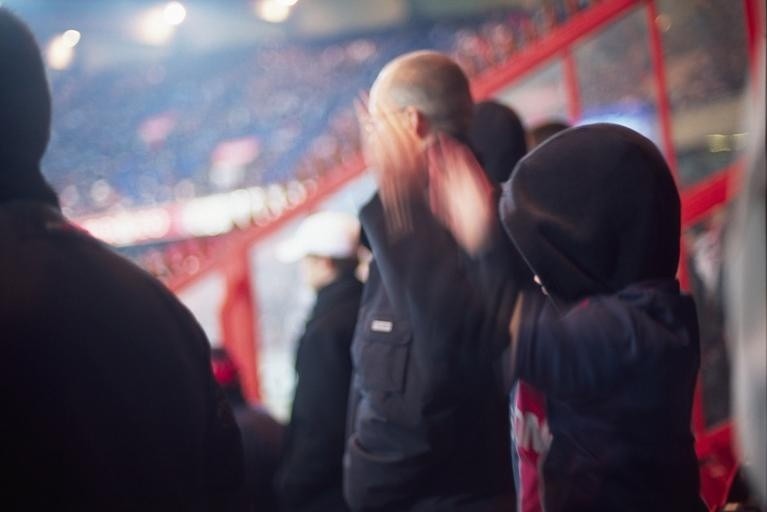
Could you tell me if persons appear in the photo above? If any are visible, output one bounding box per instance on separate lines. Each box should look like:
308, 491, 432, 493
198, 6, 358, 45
522, 117, 573, 159
342, 49, 516, 511
1, 5, 245, 511
289, 208, 367, 450
466, 94, 530, 349
354, 91, 709, 512
